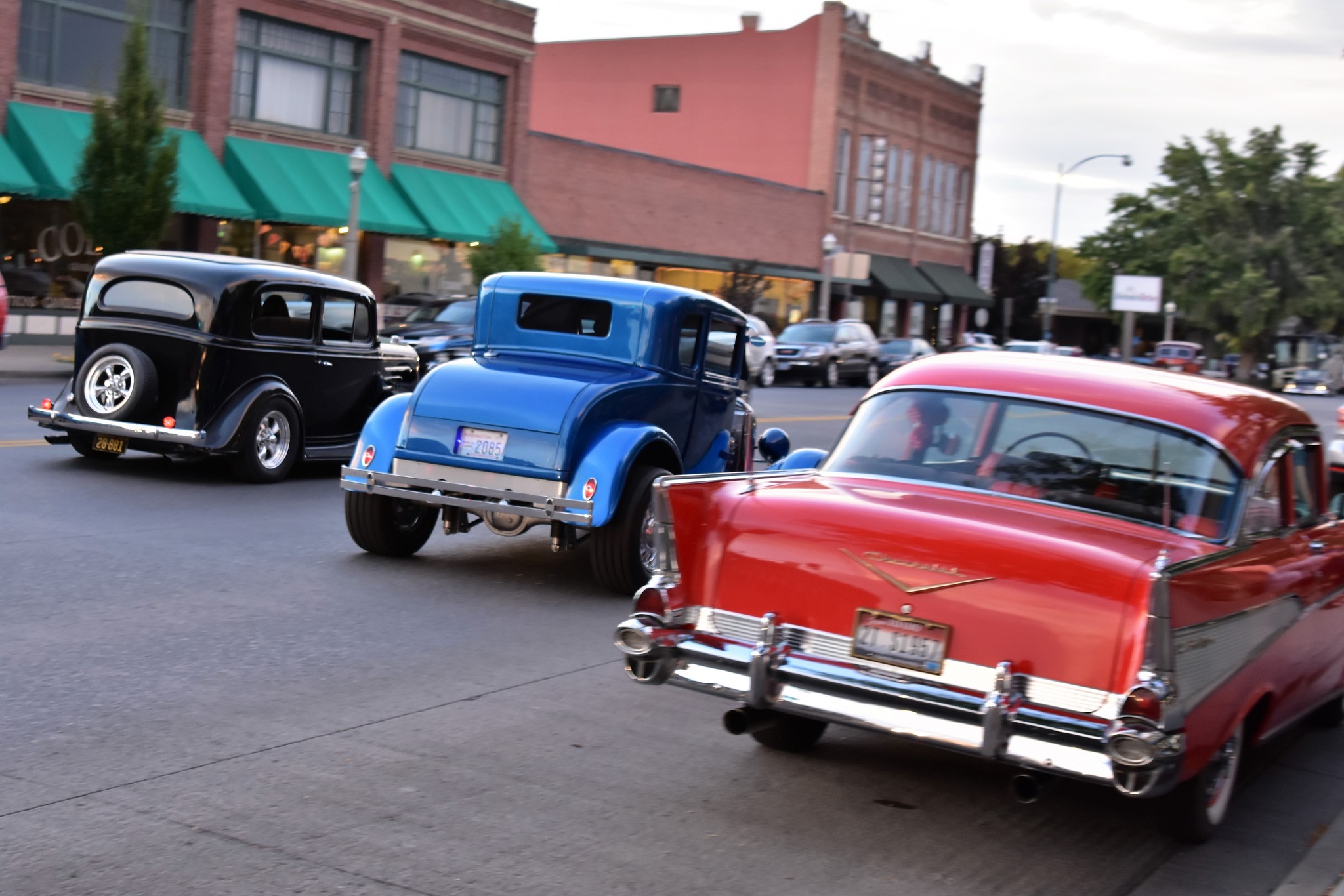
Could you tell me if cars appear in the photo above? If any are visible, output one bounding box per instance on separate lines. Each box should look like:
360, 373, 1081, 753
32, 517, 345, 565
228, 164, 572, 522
1280, 370, 1332, 397
611, 345, 1344, 847
877, 335, 938, 379
676, 313, 777, 388
948, 331, 1207, 374
27, 247, 423, 486
265, 290, 479, 380
337, 273, 834, 598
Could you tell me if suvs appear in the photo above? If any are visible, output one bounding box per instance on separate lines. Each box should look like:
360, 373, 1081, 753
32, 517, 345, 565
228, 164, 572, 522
772, 316, 883, 388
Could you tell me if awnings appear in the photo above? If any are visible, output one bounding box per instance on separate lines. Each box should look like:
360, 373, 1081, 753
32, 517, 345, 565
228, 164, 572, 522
0, 131, 40, 200
916, 259, 995, 308
224, 131, 428, 237
391, 158, 560, 254
854, 249, 942, 302
5, 102, 256, 221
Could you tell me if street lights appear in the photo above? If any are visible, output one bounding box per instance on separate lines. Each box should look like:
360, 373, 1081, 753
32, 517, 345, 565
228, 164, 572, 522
1037, 153, 1133, 341
817, 232, 837, 319
341, 146, 369, 282
